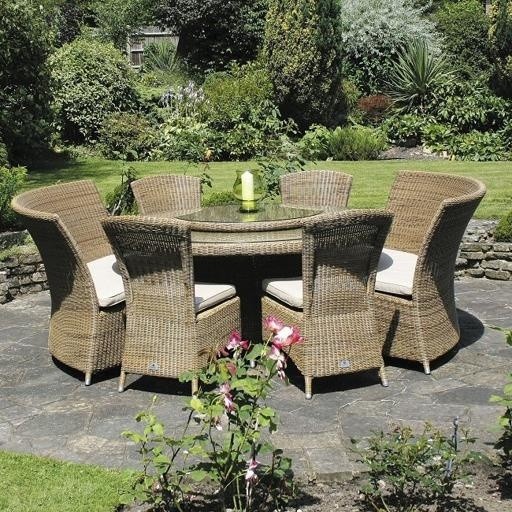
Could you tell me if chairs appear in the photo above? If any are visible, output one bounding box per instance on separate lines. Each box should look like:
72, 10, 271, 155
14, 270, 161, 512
260, 207, 395, 399
10, 179, 126, 386
373, 170, 487, 374
279, 170, 354, 207
98, 215, 242, 398
130, 174, 201, 215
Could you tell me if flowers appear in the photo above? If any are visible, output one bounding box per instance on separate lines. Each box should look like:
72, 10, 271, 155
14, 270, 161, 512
204, 316, 303, 410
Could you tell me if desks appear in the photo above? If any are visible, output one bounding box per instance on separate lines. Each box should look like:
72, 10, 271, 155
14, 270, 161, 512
161, 208, 349, 257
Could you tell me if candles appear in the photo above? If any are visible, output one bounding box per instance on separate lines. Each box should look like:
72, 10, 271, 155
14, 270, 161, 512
241, 172, 256, 211
